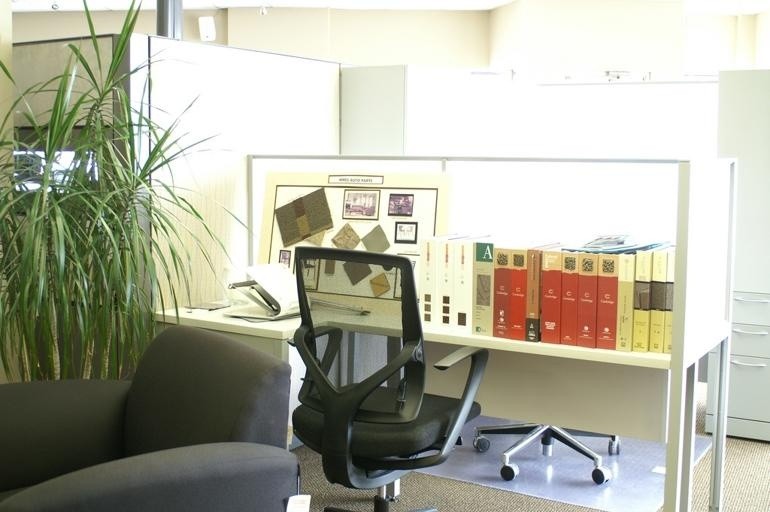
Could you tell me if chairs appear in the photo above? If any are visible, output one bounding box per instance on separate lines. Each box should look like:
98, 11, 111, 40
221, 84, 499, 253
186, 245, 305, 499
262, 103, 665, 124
472, 423, 621, 485
0, 325, 298, 512
286, 246, 488, 512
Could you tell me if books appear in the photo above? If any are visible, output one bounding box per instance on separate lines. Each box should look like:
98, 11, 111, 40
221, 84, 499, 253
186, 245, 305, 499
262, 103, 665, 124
417, 229, 672, 353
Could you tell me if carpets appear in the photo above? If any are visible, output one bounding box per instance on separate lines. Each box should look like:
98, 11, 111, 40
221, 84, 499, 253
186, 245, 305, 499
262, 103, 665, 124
411, 415, 713, 512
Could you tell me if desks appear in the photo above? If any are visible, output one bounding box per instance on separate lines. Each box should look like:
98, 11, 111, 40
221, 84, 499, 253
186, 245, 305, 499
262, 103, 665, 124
154, 154, 737, 512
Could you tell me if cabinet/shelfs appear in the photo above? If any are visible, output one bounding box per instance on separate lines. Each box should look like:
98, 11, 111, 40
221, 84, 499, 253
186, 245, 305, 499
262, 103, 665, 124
705, 69, 770, 444
